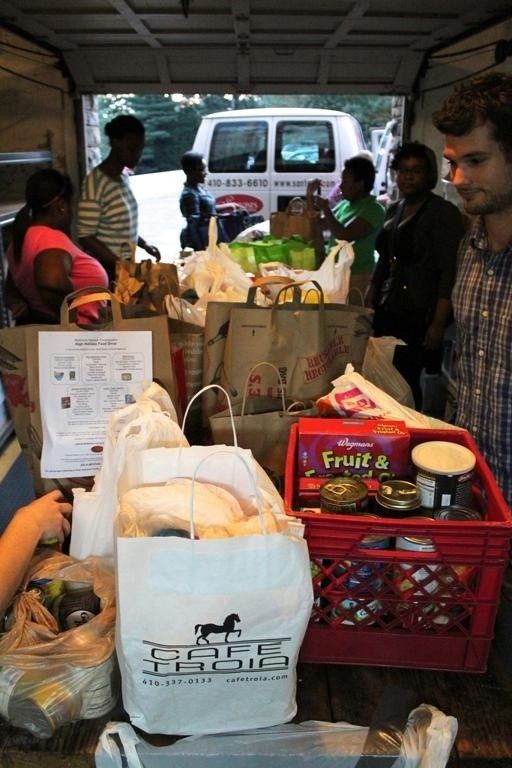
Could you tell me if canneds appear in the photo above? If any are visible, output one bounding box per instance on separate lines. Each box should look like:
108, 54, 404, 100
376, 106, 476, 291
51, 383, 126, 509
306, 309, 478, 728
319, 440, 484, 628
0, 587, 116, 740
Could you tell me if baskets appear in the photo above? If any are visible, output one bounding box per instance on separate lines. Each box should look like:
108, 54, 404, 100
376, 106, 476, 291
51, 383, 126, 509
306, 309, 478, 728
280, 411, 512, 678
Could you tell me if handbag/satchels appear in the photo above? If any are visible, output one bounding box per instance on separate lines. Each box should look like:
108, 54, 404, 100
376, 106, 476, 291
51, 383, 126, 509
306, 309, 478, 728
0, 286, 181, 501
198, 273, 380, 483
269, 193, 326, 248
113, 235, 179, 304
217, 229, 319, 270
68, 399, 191, 561
112, 374, 315, 742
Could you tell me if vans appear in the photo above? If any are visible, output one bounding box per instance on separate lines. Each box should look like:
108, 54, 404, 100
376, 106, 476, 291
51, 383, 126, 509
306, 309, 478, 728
193, 107, 367, 220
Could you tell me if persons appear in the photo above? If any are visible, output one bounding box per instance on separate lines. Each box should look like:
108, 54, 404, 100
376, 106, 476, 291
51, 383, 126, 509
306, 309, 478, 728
179, 152, 218, 250
3, 168, 110, 327
431, 71, 511, 509
305, 156, 387, 304
370, 140, 468, 414
76, 112, 163, 283
0, 489, 72, 620
